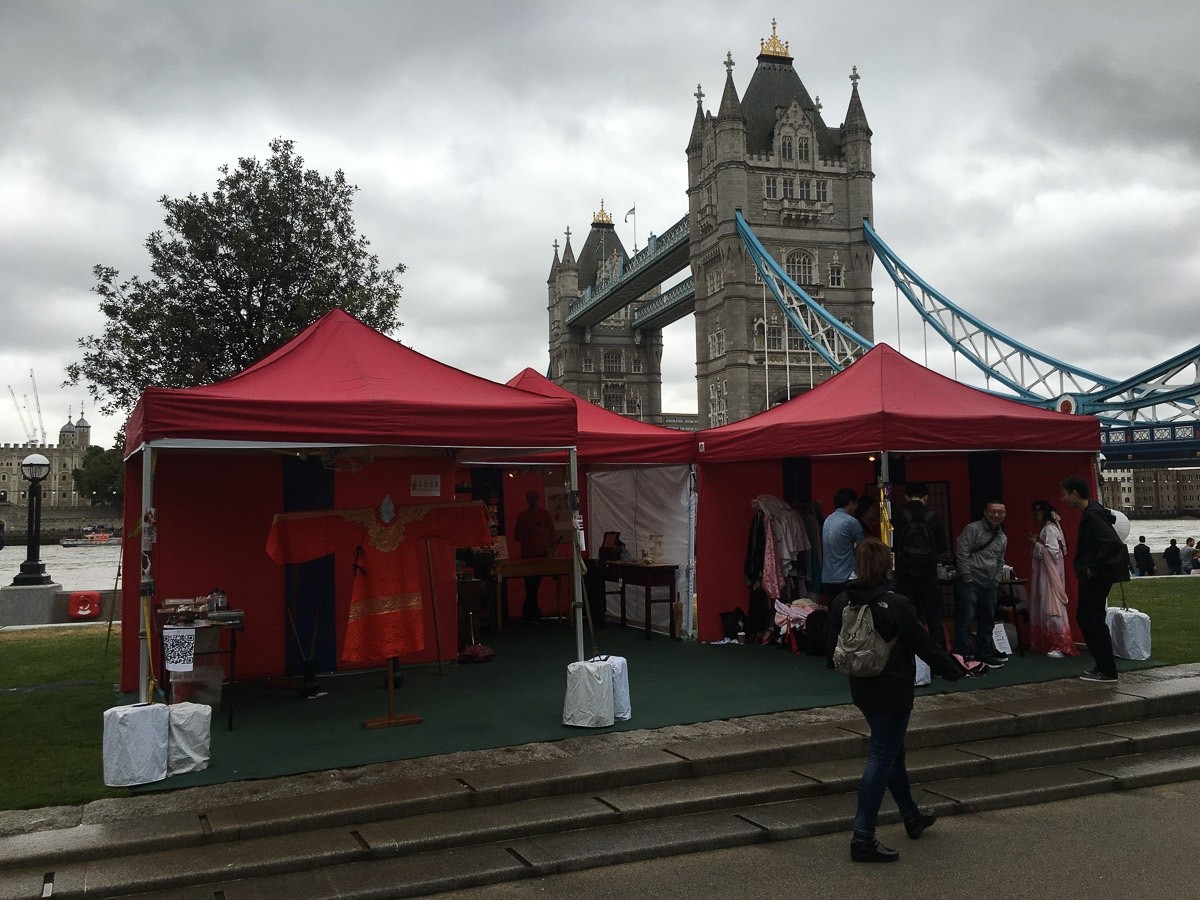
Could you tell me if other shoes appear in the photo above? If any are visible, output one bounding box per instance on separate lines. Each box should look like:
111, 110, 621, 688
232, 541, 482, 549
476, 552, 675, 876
976, 657, 1005, 669
1079, 670, 1118, 682
963, 654, 975, 663
1047, 650, 1065, 658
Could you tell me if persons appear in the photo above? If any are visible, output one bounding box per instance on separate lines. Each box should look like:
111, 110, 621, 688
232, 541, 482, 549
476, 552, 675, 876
1060, 476, 1119, 682
514, 489, 557, 622
1163, 538, 1200, 575
1026, 500, 1080, 658
831, 536, 963, 862
1134, 536, 1157, 576
822, 476, 1008, 669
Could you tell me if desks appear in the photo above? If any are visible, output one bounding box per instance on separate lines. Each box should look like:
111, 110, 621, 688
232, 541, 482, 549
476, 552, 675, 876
153, 609, 245, 731
940, 579, 1026, 656
493, 556, 572, 632
585, 558, 680, 641
459, 580, 480, 639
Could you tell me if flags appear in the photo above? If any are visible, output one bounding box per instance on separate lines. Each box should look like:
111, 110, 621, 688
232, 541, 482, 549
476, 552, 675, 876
595, 236, 603, 252
625, 208, 634, 222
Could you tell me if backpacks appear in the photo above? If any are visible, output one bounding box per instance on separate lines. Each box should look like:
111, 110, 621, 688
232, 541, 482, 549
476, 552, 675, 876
895, 506, 938, 577
833, 590, 901, 678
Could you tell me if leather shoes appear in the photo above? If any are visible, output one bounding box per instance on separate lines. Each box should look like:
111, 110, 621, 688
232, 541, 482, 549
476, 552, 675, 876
903, 808, 936, 839
851, 838, 899, 862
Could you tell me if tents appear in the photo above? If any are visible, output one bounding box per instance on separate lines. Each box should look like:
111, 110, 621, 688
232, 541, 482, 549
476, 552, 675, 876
696, 344, 1101, 646
121, 307, 697, 705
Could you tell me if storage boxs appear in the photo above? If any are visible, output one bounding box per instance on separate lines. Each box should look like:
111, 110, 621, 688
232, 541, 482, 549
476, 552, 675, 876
168, 664, 224, 716
